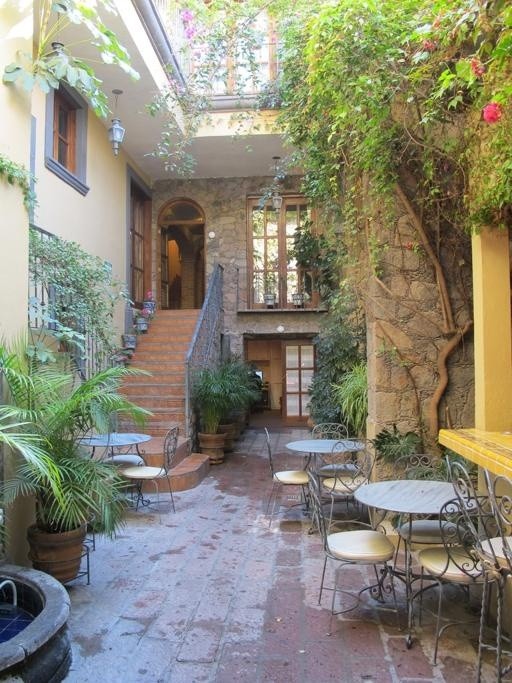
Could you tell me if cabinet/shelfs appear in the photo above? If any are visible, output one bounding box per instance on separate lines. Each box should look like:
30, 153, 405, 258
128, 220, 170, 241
253, 384, 269, 411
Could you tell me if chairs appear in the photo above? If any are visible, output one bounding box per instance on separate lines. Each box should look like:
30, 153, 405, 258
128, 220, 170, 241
285, 422, 377, 533
394, 454, 512, 683
122, 426, 180, 513
305, 472, 403, 636
265, 428, 313, 527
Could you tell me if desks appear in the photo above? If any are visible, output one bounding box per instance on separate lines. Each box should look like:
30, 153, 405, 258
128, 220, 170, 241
75, 432, 152, 511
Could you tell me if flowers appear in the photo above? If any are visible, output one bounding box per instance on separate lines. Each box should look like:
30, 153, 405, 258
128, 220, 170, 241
109, 343, 136, 362
134, 308, 150, 319
142, 289, 156, 302
126, 324, 143, 336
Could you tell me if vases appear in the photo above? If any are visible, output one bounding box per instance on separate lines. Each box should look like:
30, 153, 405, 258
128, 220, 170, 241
135, 318, 148, 330
111, 360, 126, 368
82, 472, 114, 524
122, 334, 137, 349
143, 302, 156, 314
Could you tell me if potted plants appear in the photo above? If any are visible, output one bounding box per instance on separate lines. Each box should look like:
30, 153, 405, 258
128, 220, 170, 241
187, 346, 266, 465
303, 323, 367, 431
281, 272, 306, 306
0, 315, 158, 586
252, 261, 278, 305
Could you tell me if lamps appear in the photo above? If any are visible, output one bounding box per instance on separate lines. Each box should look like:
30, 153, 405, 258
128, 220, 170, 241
270, 156, 283, 214
106, 89, 127, 158
